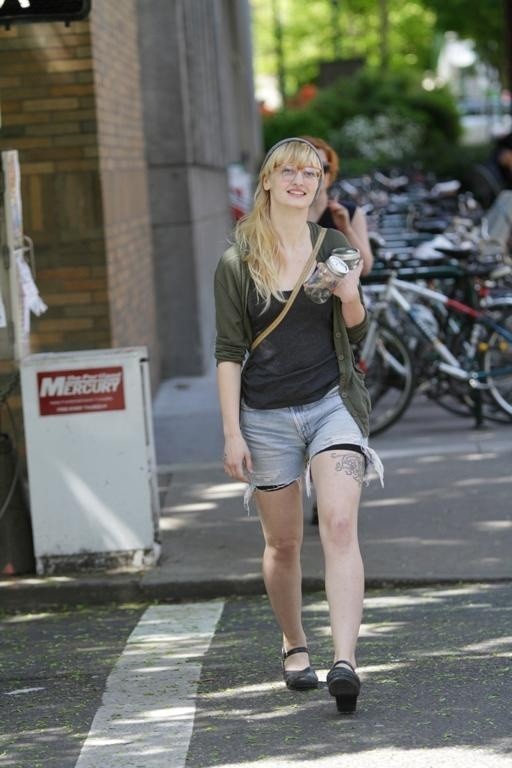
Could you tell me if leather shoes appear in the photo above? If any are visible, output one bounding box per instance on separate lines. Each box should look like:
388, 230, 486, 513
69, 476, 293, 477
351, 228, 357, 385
327, 660, 359, 711
283, 647, 317, 691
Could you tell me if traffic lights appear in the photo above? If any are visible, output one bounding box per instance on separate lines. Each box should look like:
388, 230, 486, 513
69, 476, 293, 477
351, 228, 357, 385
0, 0, 95, 30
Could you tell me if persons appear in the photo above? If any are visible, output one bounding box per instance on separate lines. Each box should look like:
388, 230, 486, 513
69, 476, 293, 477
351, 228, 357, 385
213, 138, 384, 714
484, 136, 512, 252
300, 138, 375, 527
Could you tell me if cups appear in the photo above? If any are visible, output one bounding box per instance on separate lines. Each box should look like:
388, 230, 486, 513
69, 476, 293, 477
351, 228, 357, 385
304, 256, 345, 305
330, 246, 362, 287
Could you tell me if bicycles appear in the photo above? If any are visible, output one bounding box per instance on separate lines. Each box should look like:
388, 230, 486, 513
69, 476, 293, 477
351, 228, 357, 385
318, 165, 511, 439
